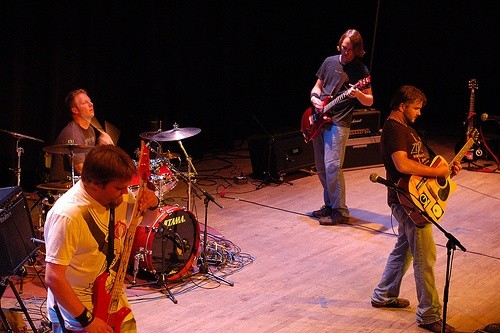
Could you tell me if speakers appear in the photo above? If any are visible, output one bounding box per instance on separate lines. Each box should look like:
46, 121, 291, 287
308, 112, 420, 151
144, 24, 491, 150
247, 131, 316, 178
341, 136, 384, 171
0, 186, 40, 276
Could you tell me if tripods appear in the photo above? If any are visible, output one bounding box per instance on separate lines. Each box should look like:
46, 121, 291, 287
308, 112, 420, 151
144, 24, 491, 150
255, 143, 293, 191
127, 141, 234, 304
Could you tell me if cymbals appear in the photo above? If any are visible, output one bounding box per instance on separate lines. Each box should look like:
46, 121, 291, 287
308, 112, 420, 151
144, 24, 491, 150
150, 127, 202, 142
3, 130, 44, 143
44, 144, 95, 154
36, 181, 77, 191
139, 131, 164, 139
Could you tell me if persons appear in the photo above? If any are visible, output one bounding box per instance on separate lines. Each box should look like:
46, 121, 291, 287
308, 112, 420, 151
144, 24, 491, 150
43, 145, 160, 333
310, 29, 374, 225
48, 89, 111, 183
371, 85, 462, 333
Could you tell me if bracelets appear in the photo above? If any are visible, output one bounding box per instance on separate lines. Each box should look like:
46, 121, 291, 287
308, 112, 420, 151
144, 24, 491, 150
75, 307, 95, 326
148, 196, 159, 210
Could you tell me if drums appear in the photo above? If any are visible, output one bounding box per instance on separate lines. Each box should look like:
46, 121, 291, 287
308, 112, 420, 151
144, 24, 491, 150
148, 159, 179, 196
40, 195, 63, 226
125, 205, 200, 281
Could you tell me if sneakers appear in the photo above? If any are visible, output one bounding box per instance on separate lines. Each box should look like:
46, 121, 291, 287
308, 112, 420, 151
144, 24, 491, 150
312, 204, 333, 218
319, 211, 349, 225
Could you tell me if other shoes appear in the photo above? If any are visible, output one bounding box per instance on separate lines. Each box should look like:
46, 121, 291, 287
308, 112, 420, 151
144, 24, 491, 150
418, 319, 452, 333
371, 297, 410, 308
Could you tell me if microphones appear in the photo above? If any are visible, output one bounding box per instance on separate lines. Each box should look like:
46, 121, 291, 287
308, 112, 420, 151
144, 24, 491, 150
370, 173, 398, 188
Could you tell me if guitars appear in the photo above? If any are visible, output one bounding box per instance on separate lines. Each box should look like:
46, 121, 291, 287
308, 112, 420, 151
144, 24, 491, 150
83, 139, 150, 333
300, 75, 371, 142
396, 128, 481, 227
455, 79, 483, 162
